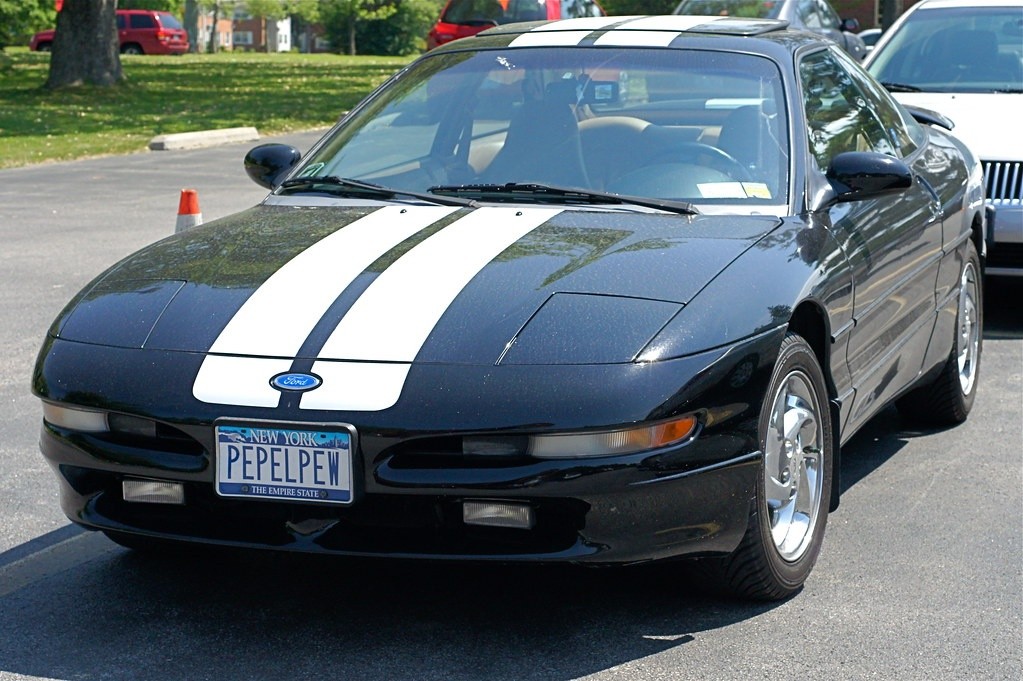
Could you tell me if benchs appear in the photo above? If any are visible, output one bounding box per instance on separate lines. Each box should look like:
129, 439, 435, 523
579, 117, 722, 186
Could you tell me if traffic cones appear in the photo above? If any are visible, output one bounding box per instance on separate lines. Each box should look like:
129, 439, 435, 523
173, 186, 201, 233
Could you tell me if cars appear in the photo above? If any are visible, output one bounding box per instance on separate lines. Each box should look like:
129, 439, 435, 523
640, 0, 869, 108
856, 28, 882, 57
853, 0, 1023, 243
426, 1, 631, 111
28, 11, 188, 58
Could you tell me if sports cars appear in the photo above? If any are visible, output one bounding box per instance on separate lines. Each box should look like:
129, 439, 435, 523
34, 17, 987, 607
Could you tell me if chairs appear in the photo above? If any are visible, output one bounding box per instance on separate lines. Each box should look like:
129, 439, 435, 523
484, 91, 590, 192
712, 103, 777, 176
948, 28, 1013, 81
